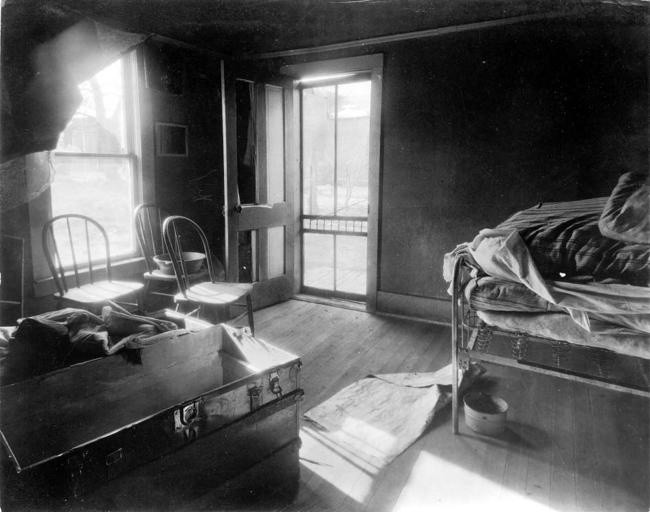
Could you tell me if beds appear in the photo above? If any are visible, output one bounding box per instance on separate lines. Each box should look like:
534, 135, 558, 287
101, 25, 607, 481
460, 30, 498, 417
443, 174, 650, 434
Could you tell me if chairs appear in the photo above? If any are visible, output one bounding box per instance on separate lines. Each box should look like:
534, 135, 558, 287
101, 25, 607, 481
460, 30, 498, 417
41, 214, 144, 319
132, 201, 209, 315
162, 215, 255, 338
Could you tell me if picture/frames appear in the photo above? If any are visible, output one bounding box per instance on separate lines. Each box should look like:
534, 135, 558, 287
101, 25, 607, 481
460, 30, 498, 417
152, 120, 191, 158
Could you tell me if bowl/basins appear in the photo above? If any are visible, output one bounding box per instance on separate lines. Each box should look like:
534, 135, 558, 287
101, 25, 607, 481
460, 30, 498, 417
153, 252, 206, 275
462, 392, 508, 437
